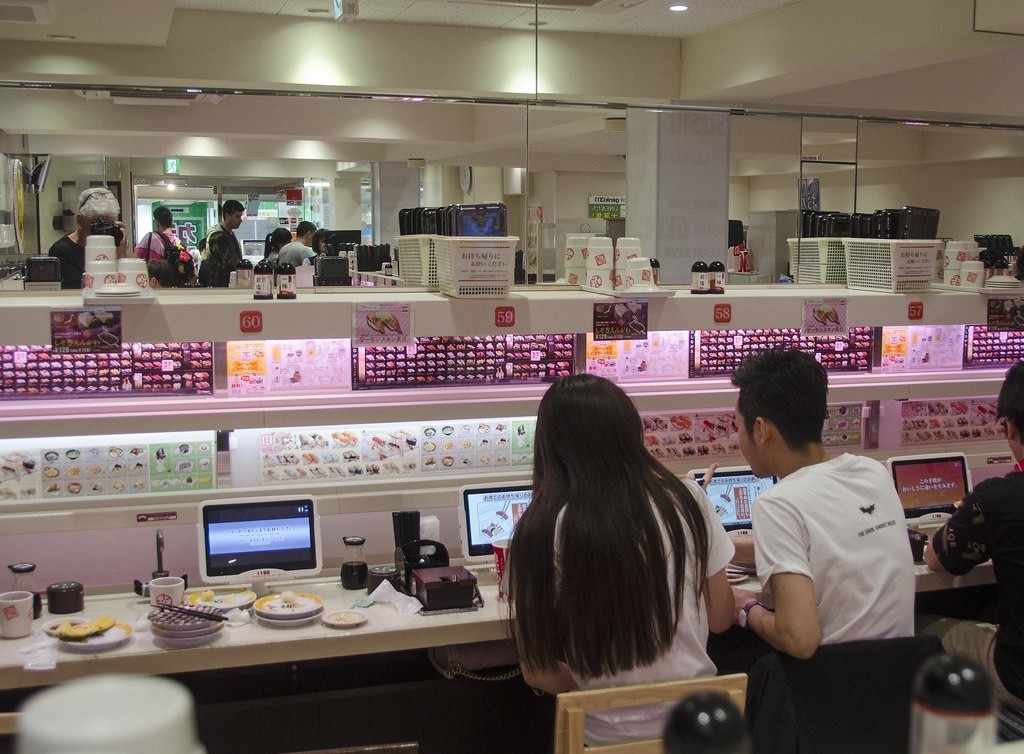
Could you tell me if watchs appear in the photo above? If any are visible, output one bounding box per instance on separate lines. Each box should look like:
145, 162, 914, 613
739, 601, 768, 629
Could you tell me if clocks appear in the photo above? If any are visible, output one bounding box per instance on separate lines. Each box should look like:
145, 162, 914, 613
459, 165, 470, 192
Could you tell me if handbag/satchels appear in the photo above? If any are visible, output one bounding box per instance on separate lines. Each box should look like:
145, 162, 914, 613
427, 639, 522, 681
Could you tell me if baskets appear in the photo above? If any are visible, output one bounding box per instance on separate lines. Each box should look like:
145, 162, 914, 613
393, 234, 438, 289
843, 237, 943, 293
431, 235, 520, 299
787, 237, 847, 283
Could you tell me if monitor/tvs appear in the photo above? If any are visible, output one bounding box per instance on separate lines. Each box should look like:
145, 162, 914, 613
458, 479, 534, 563
885, 450, 973, 529
198, 493, 323, 583
690, 466, 776, 535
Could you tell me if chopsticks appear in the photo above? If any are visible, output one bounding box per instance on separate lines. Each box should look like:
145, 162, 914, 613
149, 603, 229, 622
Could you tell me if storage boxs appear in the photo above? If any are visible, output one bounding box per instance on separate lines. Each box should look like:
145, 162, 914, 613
408, 568, 485, 612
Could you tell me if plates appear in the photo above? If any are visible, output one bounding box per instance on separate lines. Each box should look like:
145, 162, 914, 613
39, 617, 135, 651
726, 529, 756, 583
918, 513, 952, 528
322, 609, 369, 628
94, 283, 141, 298
146, 589, 326, 645
985, 276, 1021, 288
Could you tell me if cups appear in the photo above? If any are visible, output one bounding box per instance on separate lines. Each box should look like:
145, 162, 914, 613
492, 539, 516, 599
46, 581, 84, 614
367, 566, 401, 595
142, 578, 185, 611
726, 242, 750, 272
0, 590, 33, 639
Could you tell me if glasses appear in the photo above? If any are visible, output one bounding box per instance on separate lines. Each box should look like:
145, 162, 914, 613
79, 192, 115, 211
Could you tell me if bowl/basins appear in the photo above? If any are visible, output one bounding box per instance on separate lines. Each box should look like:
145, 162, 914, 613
82, 234, 151, 296
565, 235, 658, 293
339, 251, 355, 270
932, 238, 985, 288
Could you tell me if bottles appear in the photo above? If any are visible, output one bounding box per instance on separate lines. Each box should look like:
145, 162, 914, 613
690, 261, 725, 293
661, 689, 745, 754
910, 653, 997, 754
385, 264, 393, 275
236, 259, 296, 300
649, 258, 660, 286
7, 562, 42, 620
340, 536, 369, 590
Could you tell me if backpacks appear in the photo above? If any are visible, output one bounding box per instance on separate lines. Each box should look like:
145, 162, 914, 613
154, 231, 195, 288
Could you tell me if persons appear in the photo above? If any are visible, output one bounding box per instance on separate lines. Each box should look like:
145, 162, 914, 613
483, 374, 736, 754
49, 188, 128, 289
264, 221, 327, 267
134, 206, 207, 288
915, 362, 1024, 710
727, 349, 915, 677
206, 200, 245, 287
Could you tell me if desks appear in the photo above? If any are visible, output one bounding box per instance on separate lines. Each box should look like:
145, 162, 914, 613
1, 555, 992, 692
